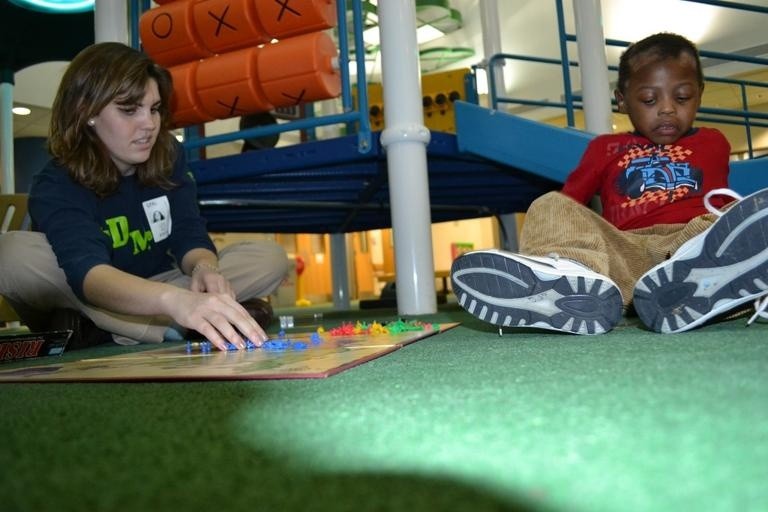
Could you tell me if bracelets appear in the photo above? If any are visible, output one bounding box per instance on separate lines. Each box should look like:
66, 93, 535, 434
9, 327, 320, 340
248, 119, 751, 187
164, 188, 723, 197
192, 262, 222, 276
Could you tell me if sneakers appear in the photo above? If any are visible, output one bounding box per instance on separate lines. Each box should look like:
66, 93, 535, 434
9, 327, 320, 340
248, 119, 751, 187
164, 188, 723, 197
633, 190, 768, 334
43, 309, 106, 351
182, 299, 274, 342
451, 249, 623, 336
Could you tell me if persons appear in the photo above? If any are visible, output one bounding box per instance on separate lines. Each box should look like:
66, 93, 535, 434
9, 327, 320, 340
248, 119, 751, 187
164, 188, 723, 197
0, 39, 294, 353
152, 210, 165, 223
448, 30, 768, 340
